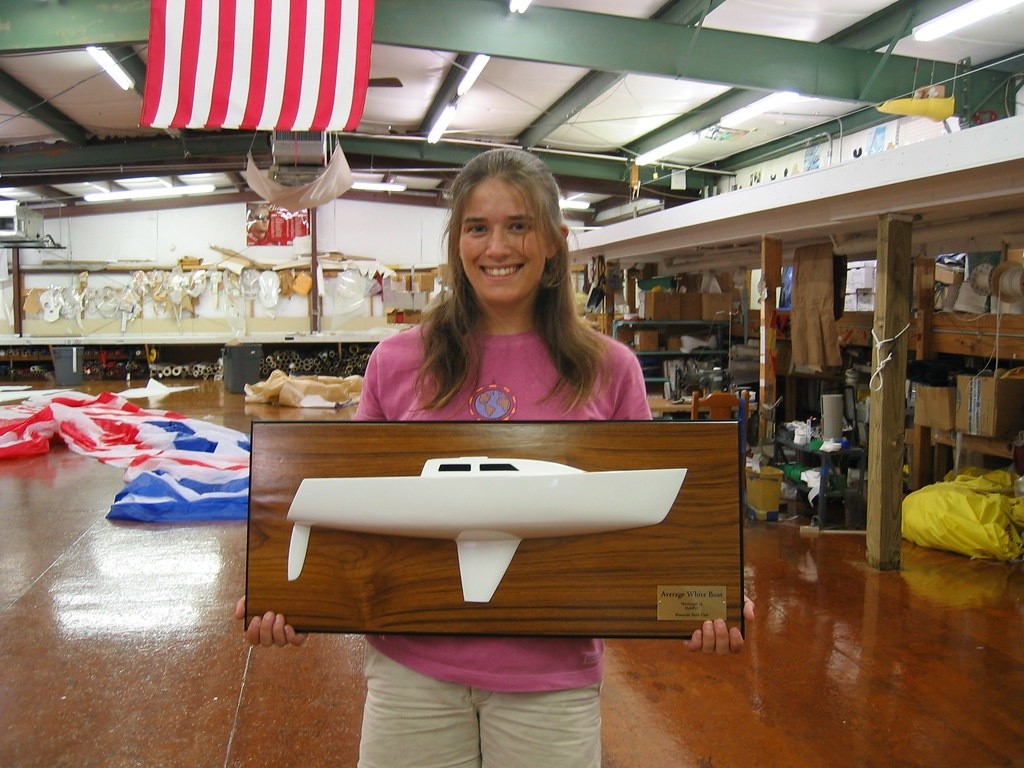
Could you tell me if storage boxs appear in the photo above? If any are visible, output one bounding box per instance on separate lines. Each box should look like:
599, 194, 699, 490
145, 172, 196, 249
384, 264, 447, 324
585, 290, 733, 352
746, 465, 784, 525
914, 374, 1024, 439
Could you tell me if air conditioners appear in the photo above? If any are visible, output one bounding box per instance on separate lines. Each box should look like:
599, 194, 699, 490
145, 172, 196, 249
270, 131, 328, 186
0, 199, 45, 247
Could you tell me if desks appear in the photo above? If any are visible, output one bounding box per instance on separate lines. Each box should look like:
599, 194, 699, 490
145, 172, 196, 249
647, 397, 757, 422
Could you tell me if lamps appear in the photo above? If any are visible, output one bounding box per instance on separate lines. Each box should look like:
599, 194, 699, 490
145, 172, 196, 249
87, 46, 135, 91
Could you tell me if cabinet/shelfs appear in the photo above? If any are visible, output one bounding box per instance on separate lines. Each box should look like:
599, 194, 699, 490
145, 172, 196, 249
774, 437, 868, 529
614, 320, 726, 383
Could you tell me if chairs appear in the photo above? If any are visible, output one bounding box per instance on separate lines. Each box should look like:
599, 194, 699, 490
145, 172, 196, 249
691, 389, 749, 446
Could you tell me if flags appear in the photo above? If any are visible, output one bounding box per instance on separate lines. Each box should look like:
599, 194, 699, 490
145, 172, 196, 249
140, 1, 374, 132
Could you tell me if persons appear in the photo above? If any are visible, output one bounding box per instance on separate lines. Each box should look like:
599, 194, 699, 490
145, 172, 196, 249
249, 207, 269, 240
234, 150, 754, 768
